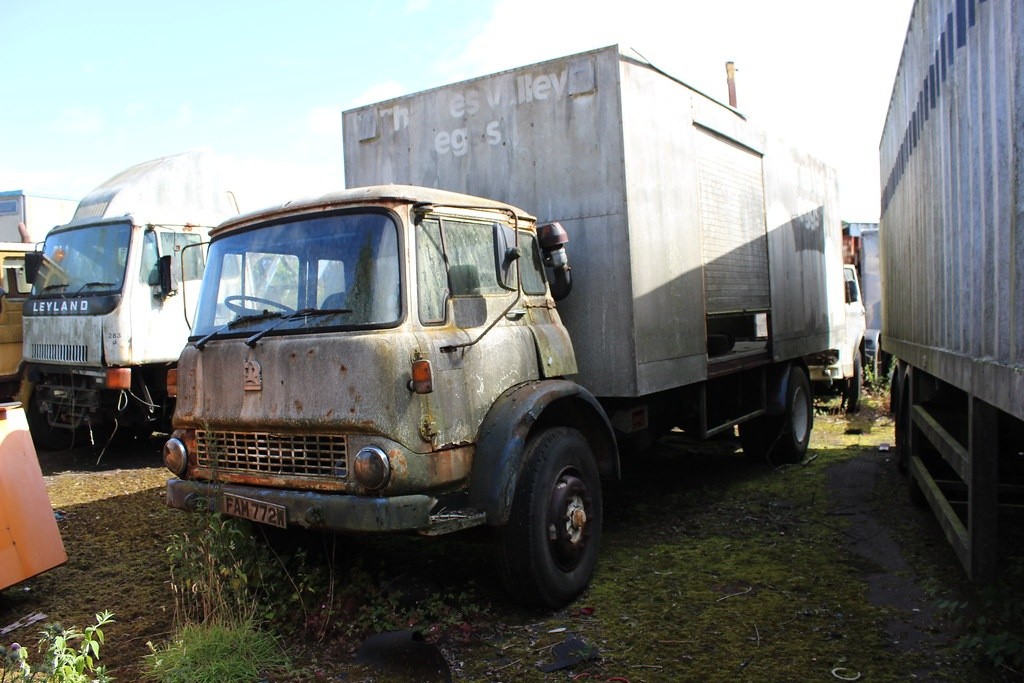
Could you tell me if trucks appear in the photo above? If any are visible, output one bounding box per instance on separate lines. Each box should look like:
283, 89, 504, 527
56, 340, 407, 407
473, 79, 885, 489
808, 218, 882, 413
163, 43, 844, 610
883, 0, 1024, 585
0, 214, 349, 592
